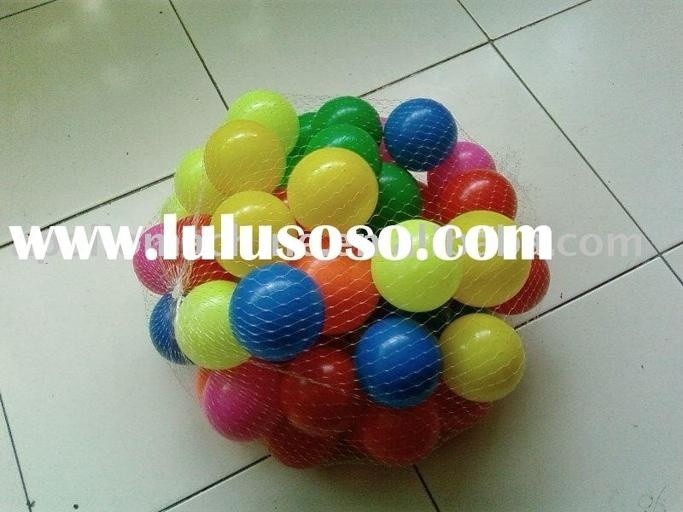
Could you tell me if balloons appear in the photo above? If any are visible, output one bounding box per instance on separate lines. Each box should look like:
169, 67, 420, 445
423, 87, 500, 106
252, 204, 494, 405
132, 85, 551, 469
